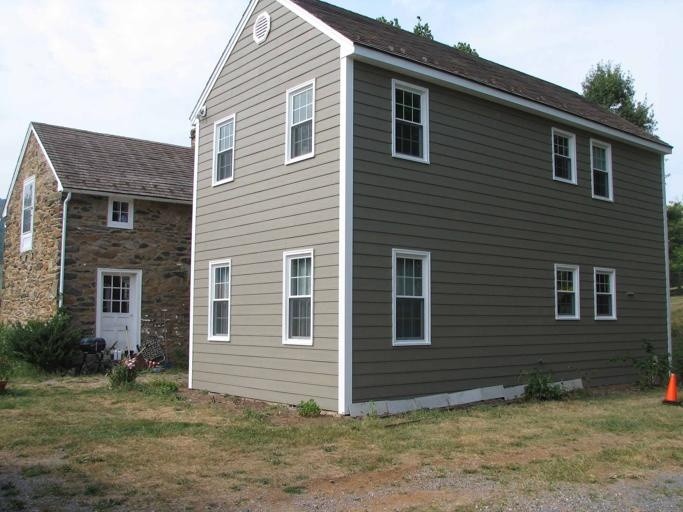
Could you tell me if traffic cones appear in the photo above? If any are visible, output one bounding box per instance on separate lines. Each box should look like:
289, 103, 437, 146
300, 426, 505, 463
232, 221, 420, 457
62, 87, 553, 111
664, 373, 678, 405
